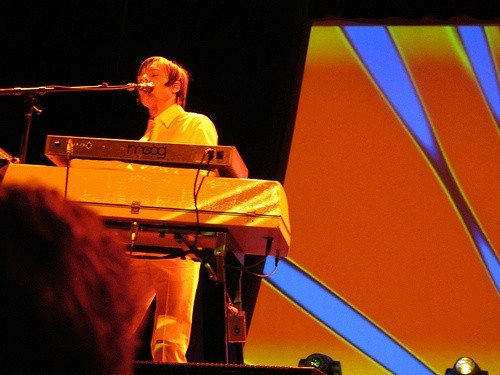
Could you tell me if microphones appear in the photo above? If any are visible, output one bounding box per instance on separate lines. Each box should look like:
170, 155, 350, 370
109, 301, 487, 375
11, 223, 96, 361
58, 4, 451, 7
125, 81, 154, 94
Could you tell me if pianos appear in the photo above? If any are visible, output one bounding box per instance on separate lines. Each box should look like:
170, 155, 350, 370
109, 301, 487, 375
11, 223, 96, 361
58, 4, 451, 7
1, 159, 291, 258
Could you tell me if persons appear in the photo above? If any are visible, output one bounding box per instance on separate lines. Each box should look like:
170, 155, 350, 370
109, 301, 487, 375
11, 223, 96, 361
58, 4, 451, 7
0, 176, 138, 375
122, 57, 219, 364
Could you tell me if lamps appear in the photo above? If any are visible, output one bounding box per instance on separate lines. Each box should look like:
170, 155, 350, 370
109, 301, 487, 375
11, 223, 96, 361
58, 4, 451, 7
445, 356, 488, 375
298, 353, 341, 375
